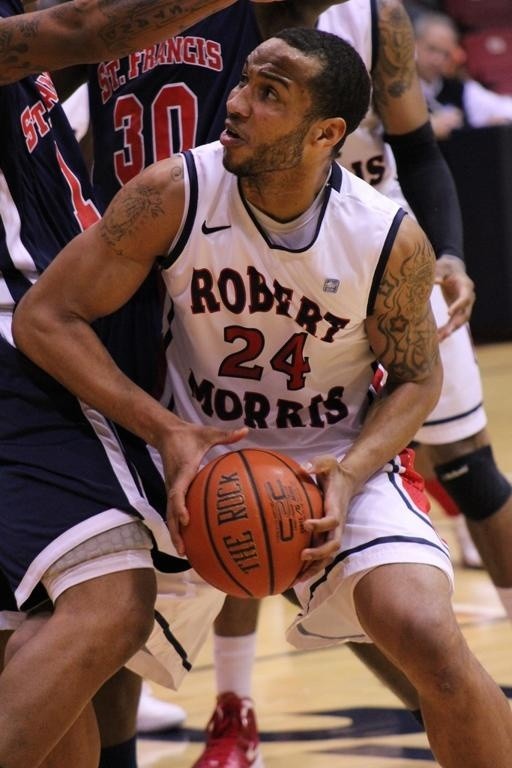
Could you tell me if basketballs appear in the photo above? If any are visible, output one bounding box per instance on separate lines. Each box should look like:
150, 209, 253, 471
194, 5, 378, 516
183, 449, 324, 598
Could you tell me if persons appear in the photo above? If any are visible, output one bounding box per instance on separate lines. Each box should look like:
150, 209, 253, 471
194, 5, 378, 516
13, 28, 510, 766
87, 0, 343, 766
318, 1, 510, 617
1, 2, 344, 768
403, 5, 510, 141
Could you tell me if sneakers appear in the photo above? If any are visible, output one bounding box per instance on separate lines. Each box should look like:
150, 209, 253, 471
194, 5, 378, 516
450, 511, 489, 571
131, 679, 188, 735
190, 687, 270, 768
